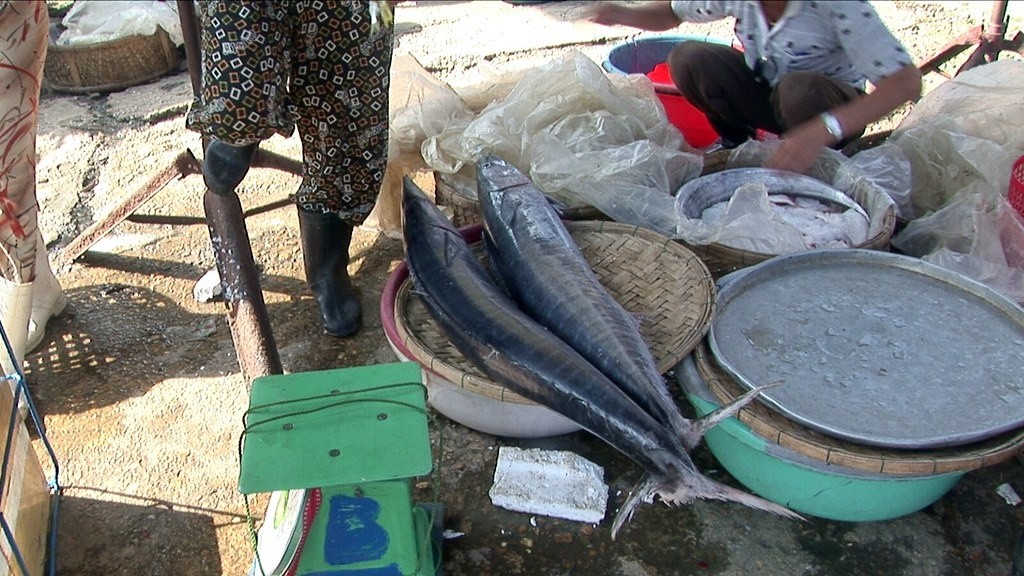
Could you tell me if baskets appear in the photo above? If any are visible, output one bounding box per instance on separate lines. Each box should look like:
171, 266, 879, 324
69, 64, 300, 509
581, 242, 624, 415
44, 22, 186, 93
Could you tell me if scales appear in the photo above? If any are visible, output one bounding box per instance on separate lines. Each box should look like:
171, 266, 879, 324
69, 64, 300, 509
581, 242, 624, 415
237, 361, 437, 576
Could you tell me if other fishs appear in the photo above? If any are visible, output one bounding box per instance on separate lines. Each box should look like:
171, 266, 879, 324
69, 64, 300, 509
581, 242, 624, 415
476, 154, 786, 541
398, 174, 811, 522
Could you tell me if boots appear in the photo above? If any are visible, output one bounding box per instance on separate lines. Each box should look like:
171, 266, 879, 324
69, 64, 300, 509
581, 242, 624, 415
0, 275, 37, 420
297, 205, 362, 336
25, 228, 67, 354
203, 138, 262, 196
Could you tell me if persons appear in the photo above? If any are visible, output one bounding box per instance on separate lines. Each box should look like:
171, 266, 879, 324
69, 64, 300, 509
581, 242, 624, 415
587, 0, 922, 175
0, 0, 69, 422
185, 0, 394, 339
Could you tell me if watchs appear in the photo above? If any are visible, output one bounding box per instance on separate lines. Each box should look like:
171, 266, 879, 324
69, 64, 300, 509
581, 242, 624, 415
819, 111, 844, 142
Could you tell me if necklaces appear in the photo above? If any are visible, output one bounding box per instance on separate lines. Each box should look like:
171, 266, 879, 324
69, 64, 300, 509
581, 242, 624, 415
767, 18, 777, 27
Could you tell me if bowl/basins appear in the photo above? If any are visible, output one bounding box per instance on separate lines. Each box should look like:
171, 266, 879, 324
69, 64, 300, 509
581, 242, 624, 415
675, 353, 970, 522
380, 220, 582, 436
601, 33, 731, 149
